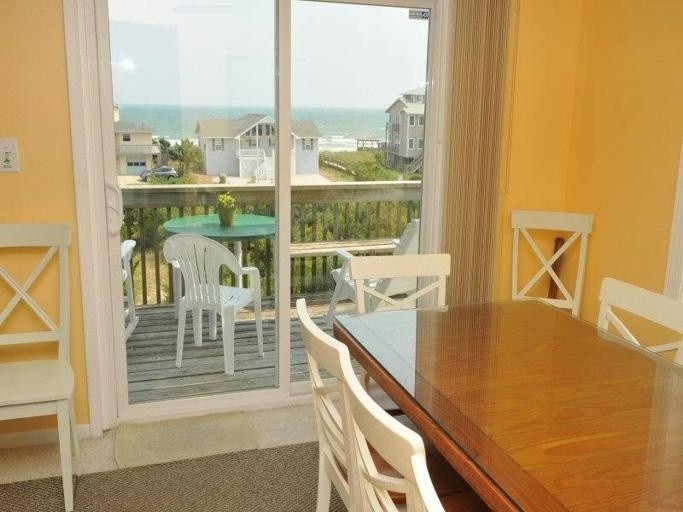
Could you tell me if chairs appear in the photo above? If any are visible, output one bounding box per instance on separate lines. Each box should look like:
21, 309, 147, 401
162, 232, 266, 376
349, 253, 451, 402
0, 225, 81, 511
508, 206, 682, 368
296, 297, 446, 511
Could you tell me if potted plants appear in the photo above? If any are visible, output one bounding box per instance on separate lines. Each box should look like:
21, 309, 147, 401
213, 191, 239, 226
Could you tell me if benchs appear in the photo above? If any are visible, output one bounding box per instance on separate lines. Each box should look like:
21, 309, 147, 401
290, 239, 398, 258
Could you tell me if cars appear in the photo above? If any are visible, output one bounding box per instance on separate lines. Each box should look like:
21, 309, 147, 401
139, 165, 175, 181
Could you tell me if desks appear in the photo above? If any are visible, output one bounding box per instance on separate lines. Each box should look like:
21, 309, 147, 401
331, 300, 683, 512
158, 212, 276, 286
